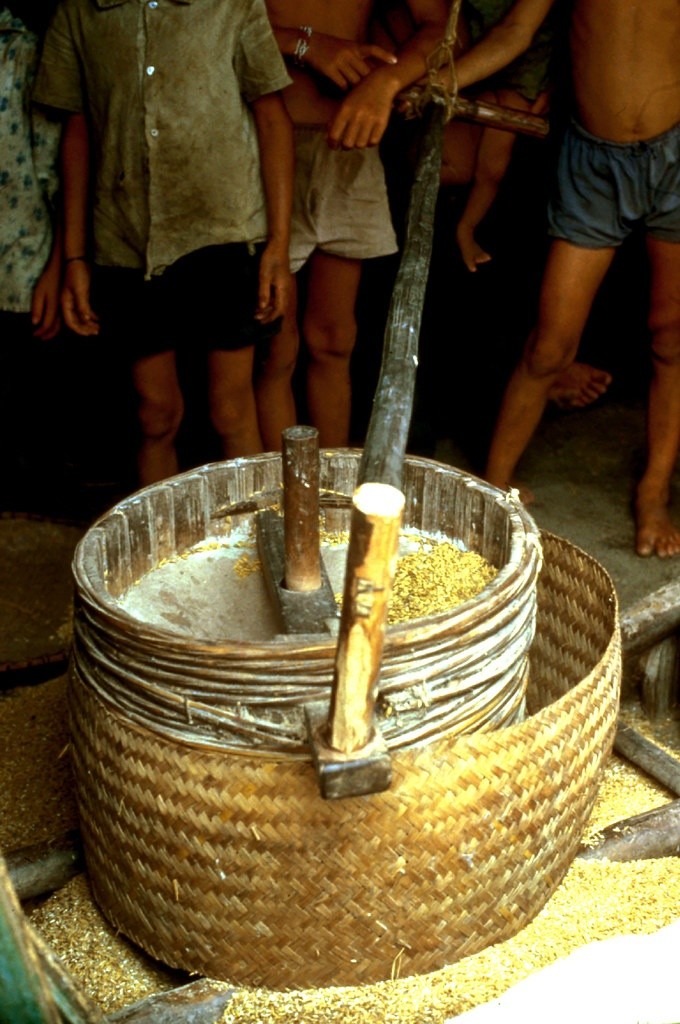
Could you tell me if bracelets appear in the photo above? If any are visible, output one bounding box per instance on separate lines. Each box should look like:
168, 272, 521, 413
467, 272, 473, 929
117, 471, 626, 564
65, 256, 87, 265
293, 24, 312, 67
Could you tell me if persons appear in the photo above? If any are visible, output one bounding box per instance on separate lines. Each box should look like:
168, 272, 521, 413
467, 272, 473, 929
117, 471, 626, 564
1, 0, 63, 467
404, 1, 680, 559
372, 0, 613, 409
31, 0, 296, 488
258, 0, 469, 453
